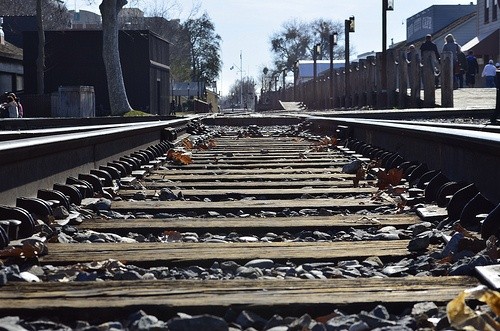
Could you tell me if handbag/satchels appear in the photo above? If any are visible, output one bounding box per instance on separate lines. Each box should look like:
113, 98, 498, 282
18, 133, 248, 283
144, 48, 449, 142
453, 62, 460, 74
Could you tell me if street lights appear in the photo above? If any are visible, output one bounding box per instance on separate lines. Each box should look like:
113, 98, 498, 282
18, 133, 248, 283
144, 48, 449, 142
381, 0, 395, 110
260, 87, 265, 104
229, 49, 244, 108
282, 67, 288, 102
313, 42, 321, 110
275, 75, 279, 109
329, 30, 339, 109
293, 59, 299, 102
268, 79, 273, 110
344, 14, 356, 112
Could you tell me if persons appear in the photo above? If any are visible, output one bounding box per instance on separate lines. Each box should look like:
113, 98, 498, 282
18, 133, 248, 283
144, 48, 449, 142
407, 33, 500, 90
4, 93, 23, 118
170, 98, 176, 115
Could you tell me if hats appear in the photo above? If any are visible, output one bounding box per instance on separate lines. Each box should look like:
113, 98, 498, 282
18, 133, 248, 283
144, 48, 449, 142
496, 63, 500, 66
409, 45, 415, 49
425, 34, 432, 39
489, 60, 493, 63
